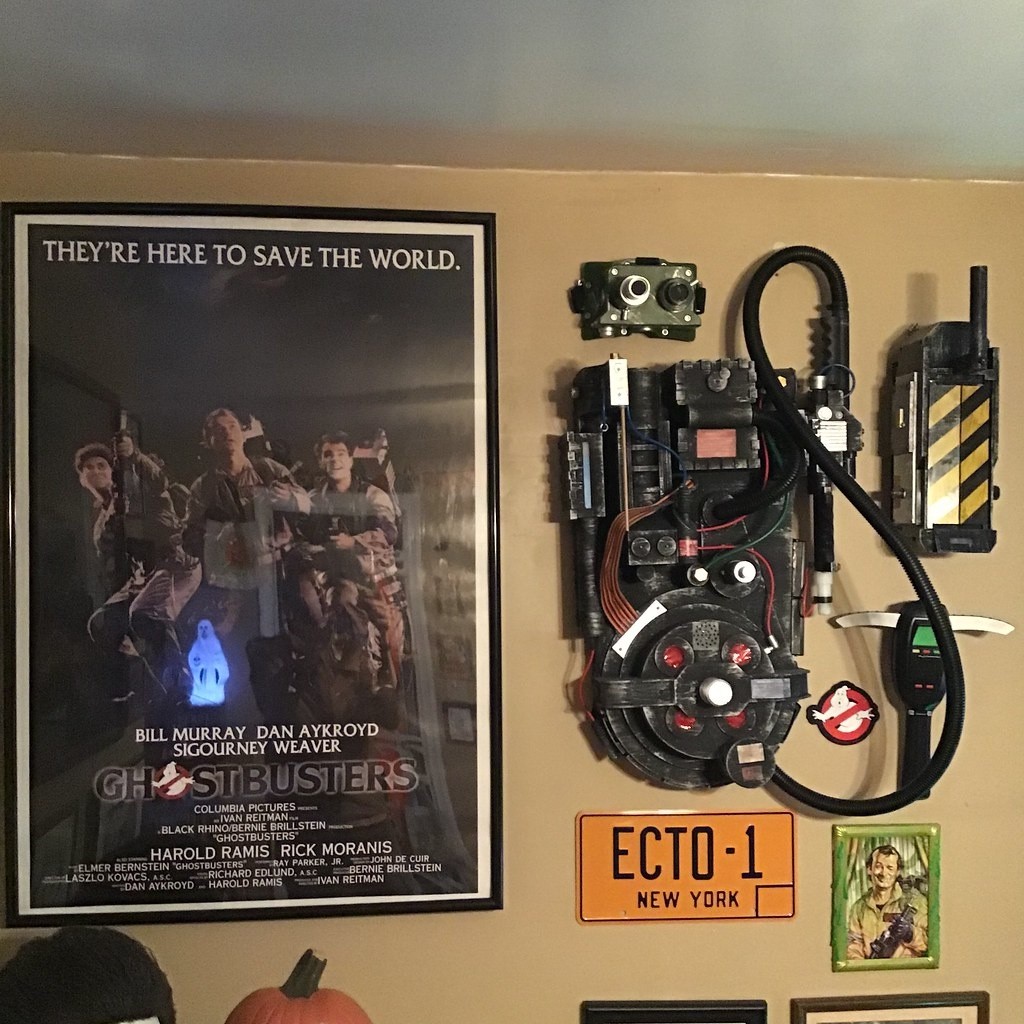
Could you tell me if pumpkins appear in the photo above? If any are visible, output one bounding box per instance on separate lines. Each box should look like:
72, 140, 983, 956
225, 950, 373, 1024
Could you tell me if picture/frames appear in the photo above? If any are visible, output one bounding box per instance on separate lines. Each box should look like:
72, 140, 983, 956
1, 200, 505, 929
832, 826, 940, 973
790, 990, 990, 1024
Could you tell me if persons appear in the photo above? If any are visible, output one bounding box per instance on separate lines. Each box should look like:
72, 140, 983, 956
0, 926, 176, 1024
74, 409, 405, 747
